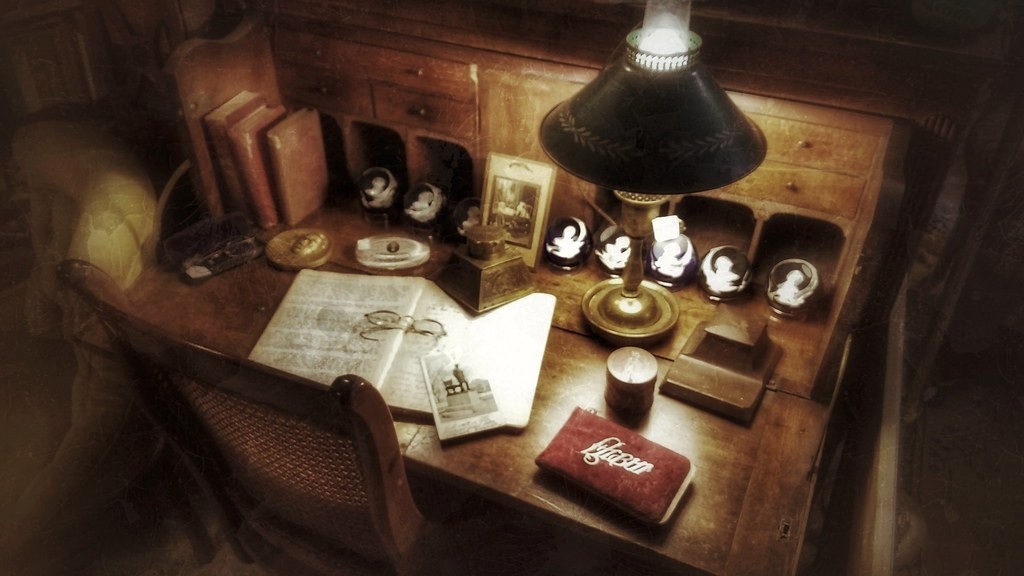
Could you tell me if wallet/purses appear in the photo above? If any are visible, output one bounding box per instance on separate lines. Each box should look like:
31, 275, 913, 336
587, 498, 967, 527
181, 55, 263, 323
535, 406, 691, 523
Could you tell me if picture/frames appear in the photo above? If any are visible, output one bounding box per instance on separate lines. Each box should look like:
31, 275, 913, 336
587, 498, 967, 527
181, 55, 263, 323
482, 150, 560, 272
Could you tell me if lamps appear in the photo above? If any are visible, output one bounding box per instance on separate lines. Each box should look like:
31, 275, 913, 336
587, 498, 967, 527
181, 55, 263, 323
538, 0, 770, 348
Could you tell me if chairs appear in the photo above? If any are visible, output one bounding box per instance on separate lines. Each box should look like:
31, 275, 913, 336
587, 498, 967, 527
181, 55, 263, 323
60, 261, 549, 576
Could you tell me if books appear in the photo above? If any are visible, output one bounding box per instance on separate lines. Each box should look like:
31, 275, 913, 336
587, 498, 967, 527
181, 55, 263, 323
246, 266, 557, 437
202, 88, 329, 230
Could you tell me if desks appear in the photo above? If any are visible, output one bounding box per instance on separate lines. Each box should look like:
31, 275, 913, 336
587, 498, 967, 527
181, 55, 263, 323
72, 0, 915, 575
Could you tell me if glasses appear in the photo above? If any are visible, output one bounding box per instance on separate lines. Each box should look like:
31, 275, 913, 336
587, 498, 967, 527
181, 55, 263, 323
181, 235, 256, 274
362, 309, 446, 339
